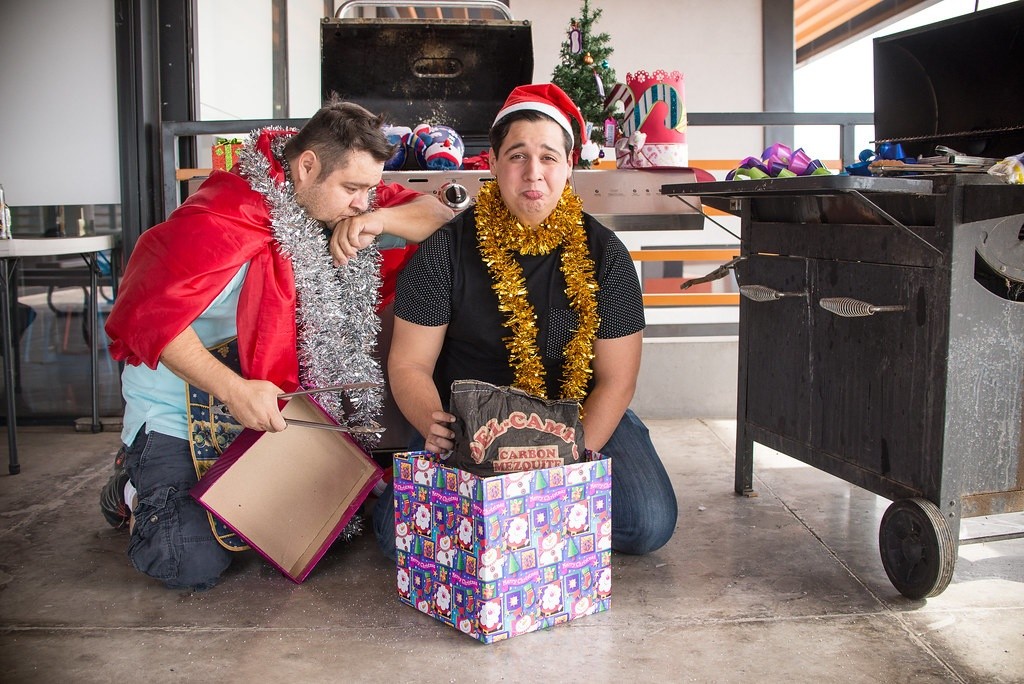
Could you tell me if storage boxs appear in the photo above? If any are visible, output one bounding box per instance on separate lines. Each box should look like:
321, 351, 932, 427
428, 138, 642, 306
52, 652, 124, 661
392, 448, 614, 644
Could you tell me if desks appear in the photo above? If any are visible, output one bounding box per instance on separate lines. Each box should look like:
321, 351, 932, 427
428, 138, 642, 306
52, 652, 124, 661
0, 230, 119, 476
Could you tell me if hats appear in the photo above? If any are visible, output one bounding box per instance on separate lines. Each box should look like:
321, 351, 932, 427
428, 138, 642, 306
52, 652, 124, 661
494, 84, 586, 151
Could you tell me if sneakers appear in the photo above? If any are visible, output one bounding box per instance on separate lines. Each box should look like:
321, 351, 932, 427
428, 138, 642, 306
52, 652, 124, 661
99, 445, 129, 530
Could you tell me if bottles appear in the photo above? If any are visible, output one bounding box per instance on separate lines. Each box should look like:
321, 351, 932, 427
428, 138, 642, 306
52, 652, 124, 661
0, 183, 11, 240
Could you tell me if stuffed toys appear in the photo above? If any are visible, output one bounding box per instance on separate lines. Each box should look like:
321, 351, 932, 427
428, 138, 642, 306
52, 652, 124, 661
380, 125, 466, 170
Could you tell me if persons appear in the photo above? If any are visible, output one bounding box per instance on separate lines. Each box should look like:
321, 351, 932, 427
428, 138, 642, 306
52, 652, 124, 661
374, 81, 677, 567
99, 90, 454, 595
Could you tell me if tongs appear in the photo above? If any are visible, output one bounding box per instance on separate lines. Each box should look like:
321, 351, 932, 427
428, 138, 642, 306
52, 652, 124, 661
917, 154, 1001, 165
936, 145, 966, 155
209, 382, 386, 432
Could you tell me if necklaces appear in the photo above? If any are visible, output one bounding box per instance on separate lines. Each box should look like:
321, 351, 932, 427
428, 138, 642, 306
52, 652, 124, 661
473, 179, 601, 420
238, 124, 386, 454
